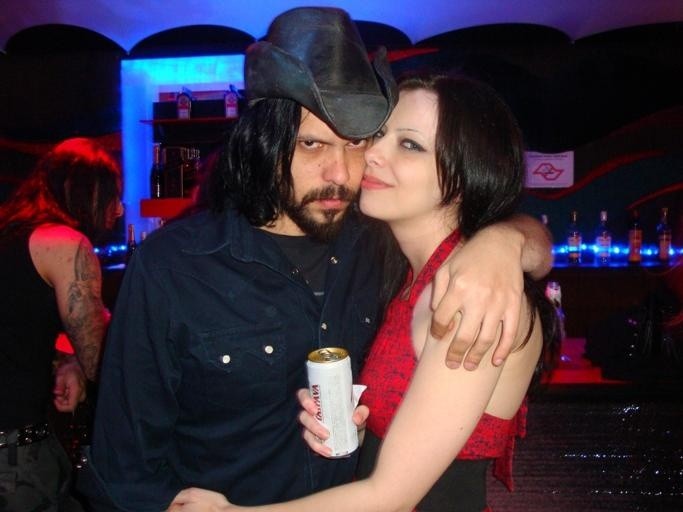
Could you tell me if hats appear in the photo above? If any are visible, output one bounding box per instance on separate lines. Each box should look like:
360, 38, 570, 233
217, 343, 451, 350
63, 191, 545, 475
244, 6, 398, 142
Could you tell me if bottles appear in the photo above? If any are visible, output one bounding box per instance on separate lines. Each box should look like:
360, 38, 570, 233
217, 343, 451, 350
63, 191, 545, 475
176, 88, 192, 119
567, 208, 671, 264
223, 84, 239, 119
538, 279, 565, 371
126, 224, 147, 266
150, 141, 166, 198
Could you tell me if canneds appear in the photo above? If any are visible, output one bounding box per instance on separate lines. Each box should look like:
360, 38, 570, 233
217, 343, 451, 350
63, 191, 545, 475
305, 347, 359, 459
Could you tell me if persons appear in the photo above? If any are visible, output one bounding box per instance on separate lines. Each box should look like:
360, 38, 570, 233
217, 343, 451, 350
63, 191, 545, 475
168, 65, 564, 512
643, 277, 683, 323
0, 134, 124, 512
88, 5, 555, 511
